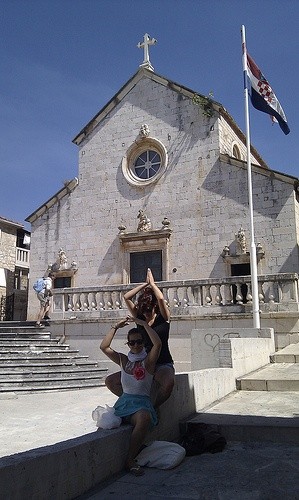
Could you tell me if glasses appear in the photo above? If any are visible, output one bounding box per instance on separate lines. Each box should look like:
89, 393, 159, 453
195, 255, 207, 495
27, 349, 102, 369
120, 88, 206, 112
128, 340, 143, 346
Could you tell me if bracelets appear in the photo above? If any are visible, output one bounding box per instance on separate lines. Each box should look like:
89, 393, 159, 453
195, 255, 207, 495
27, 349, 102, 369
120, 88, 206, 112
111, 323, 119, 330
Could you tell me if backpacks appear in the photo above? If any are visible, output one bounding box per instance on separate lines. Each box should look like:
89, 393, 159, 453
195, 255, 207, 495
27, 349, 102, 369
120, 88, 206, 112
33, 278, 50, 292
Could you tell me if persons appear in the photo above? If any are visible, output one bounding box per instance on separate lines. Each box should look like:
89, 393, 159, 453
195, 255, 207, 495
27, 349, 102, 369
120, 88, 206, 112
105, 268, 175, 425
36, 272, 56, 328
100, 314, 162, 477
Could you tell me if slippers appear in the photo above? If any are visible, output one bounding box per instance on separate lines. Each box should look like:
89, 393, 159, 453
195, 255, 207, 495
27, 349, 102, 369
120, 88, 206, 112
129, 466, 144, 476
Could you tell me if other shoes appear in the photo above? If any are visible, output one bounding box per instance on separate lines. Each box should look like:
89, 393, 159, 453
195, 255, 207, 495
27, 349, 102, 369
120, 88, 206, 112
36, 323, 43, 327
43, 316, 50, 319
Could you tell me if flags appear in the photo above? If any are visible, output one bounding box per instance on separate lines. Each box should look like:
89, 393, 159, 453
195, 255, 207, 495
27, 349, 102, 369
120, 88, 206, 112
247, 56, 290, 136
